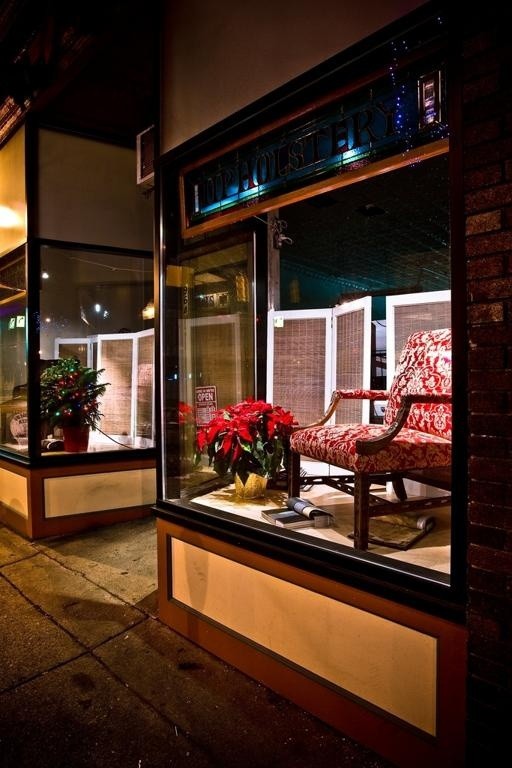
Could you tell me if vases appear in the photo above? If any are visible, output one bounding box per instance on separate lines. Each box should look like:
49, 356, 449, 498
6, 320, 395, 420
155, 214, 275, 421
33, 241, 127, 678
230, 468, 269, 500
61, 423, 92, 454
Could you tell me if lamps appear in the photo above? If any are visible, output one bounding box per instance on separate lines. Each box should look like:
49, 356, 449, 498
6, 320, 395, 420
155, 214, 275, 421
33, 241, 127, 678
271, 215, 293, 250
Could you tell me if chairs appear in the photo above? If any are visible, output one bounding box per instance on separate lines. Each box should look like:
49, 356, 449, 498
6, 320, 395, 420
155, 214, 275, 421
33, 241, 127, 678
284, 322, 454, 554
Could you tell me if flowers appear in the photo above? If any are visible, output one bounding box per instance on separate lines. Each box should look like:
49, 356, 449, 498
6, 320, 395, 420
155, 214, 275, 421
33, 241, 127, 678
194, 397, 300, 487
37, 356, 110, 428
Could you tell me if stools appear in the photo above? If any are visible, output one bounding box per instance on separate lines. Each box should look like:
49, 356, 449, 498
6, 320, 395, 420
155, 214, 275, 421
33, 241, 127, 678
1, 398, 27, 445
